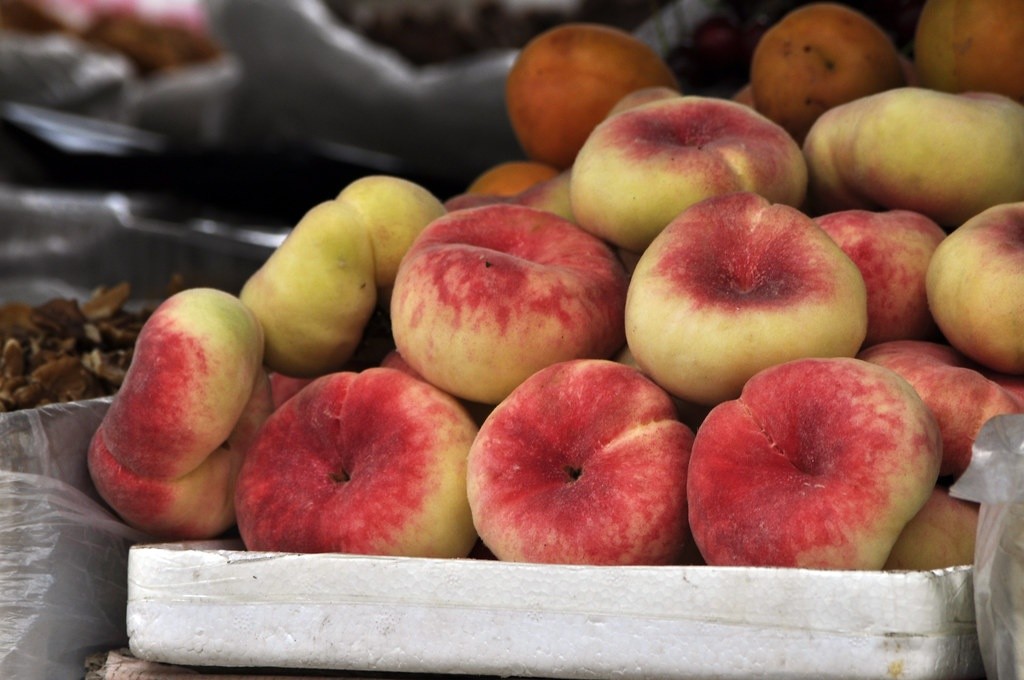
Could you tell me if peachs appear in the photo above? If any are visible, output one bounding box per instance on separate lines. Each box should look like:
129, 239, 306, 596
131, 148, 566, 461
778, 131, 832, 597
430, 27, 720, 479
88, 0, 1024, 571
